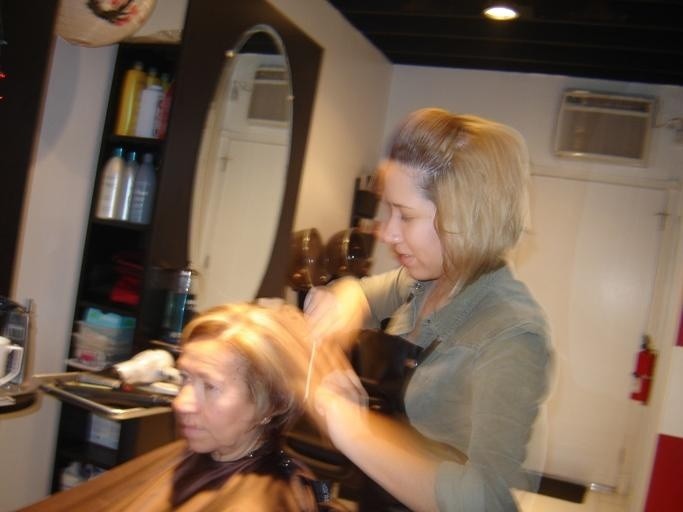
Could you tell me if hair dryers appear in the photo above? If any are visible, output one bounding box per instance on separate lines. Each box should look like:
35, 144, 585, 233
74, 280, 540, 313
111, 350, 193, 389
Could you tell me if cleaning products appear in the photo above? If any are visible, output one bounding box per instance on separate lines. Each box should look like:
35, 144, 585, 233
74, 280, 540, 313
112, 63, 168, 139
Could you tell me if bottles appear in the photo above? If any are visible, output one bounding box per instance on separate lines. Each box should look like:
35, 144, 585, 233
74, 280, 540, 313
162, 271, 192, 343
95, 63, 171, 225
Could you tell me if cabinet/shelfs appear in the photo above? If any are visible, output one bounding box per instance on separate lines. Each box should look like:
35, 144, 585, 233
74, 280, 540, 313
51, 39, 181, 496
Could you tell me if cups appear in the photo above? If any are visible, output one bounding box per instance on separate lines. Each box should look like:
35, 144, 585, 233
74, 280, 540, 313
0, 337, 24, 387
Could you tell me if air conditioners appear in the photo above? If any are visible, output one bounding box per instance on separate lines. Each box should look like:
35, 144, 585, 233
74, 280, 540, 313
554, 89, 656, 168
246, 64, 291, 128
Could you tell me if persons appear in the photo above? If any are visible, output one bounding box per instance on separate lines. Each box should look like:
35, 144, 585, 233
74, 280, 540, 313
17, 301, 373, 511
255, 105, 552, 511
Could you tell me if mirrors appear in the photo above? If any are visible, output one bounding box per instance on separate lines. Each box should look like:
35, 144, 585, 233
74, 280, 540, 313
186, 21, 295, 312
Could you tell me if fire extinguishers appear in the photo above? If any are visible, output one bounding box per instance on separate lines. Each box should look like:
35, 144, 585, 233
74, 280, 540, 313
629, 334, 656, 402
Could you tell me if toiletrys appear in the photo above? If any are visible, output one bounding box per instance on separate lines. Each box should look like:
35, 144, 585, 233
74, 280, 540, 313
96, 146, 157, 222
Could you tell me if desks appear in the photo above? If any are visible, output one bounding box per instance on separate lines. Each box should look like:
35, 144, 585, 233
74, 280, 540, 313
0, 388, 43, 420
40, 379, 177, 420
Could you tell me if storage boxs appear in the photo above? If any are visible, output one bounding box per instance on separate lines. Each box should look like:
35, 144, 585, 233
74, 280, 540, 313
74, 308, 137, 368
